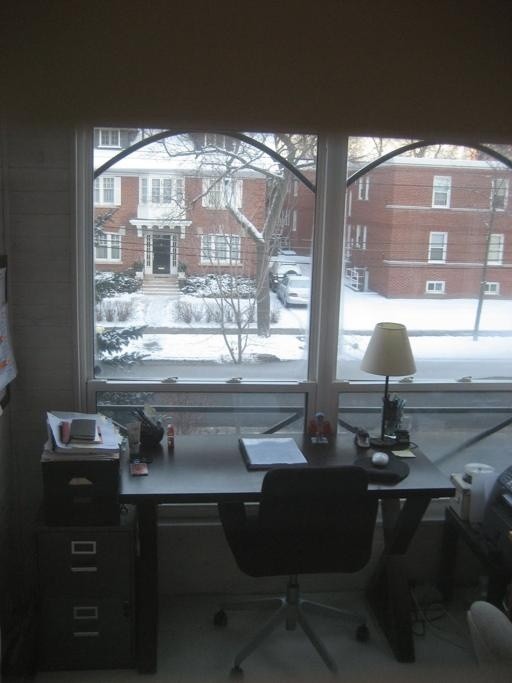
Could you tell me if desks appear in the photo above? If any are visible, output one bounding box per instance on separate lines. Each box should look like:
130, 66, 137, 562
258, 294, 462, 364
122, 428, 455, 672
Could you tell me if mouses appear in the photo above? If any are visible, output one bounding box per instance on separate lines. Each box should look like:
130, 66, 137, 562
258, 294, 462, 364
371, 451, 391, 467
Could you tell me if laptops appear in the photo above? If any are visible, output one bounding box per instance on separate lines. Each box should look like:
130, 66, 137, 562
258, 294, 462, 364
237, 437, 308, 471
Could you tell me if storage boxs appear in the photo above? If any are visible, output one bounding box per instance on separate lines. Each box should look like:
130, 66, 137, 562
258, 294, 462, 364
43, 436, 131, 526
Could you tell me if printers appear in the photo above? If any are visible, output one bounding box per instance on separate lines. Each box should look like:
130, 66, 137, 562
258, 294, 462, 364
479, 465, 512, 560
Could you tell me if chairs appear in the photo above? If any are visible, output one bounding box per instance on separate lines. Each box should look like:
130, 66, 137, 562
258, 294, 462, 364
213, 463, 378, 678
469, 599, 511, 683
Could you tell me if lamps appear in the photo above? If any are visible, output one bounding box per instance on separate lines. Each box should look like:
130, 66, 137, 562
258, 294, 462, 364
361, 322, 415, 451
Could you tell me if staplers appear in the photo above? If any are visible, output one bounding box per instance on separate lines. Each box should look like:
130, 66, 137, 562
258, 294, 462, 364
356, 426, 370, 447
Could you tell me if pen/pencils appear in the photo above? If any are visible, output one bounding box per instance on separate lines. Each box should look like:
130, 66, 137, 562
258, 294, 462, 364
131, 408, 155, 430
385, 393, 406, 435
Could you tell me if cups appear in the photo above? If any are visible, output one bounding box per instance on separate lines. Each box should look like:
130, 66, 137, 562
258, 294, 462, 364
382, 416, 400, 441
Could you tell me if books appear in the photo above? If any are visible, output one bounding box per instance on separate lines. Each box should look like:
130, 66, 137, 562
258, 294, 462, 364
62, 421, 104, 444
70, 418, 97, 440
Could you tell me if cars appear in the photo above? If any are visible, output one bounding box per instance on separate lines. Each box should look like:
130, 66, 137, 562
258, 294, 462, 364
269, 261, 312, 308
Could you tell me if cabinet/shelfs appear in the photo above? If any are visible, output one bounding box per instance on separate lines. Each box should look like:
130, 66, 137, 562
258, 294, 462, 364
34, 508, 137, 669
